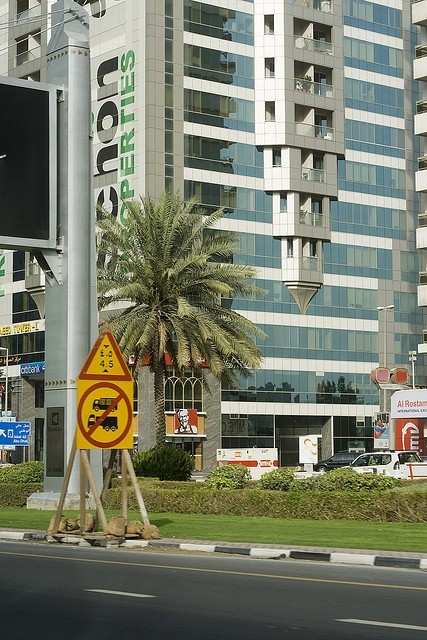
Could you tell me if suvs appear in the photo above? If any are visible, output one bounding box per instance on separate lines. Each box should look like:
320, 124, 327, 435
343, 450, 422, 479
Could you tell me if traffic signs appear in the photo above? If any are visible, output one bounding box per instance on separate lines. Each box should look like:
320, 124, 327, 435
0, 422, 31, 446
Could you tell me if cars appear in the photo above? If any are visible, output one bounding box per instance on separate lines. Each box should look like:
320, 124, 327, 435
313, 452, 362, 472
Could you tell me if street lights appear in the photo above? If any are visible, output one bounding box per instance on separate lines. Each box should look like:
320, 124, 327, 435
409, 351, 416, 390
0, 347, 8, 417
377, 304, 394, 452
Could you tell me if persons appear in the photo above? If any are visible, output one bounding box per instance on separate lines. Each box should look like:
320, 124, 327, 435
174, 409, 197, 434
